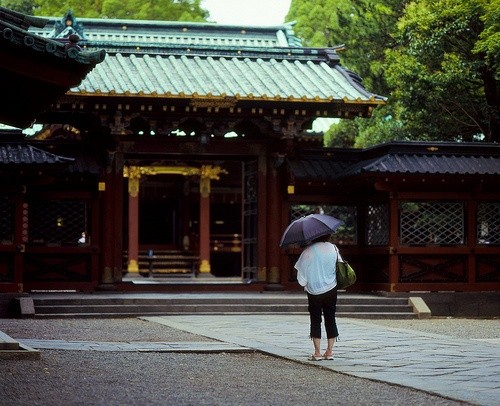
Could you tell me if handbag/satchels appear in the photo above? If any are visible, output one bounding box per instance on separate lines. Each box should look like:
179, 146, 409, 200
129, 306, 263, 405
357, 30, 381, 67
337, 261, 356, 291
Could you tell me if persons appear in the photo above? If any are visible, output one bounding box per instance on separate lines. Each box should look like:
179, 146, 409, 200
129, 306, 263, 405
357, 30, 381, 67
296, 228, 344, 361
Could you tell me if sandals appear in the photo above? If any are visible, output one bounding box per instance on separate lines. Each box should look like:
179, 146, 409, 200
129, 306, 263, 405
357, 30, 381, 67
322, 353, 334, 360
308, 354, 322, 361
